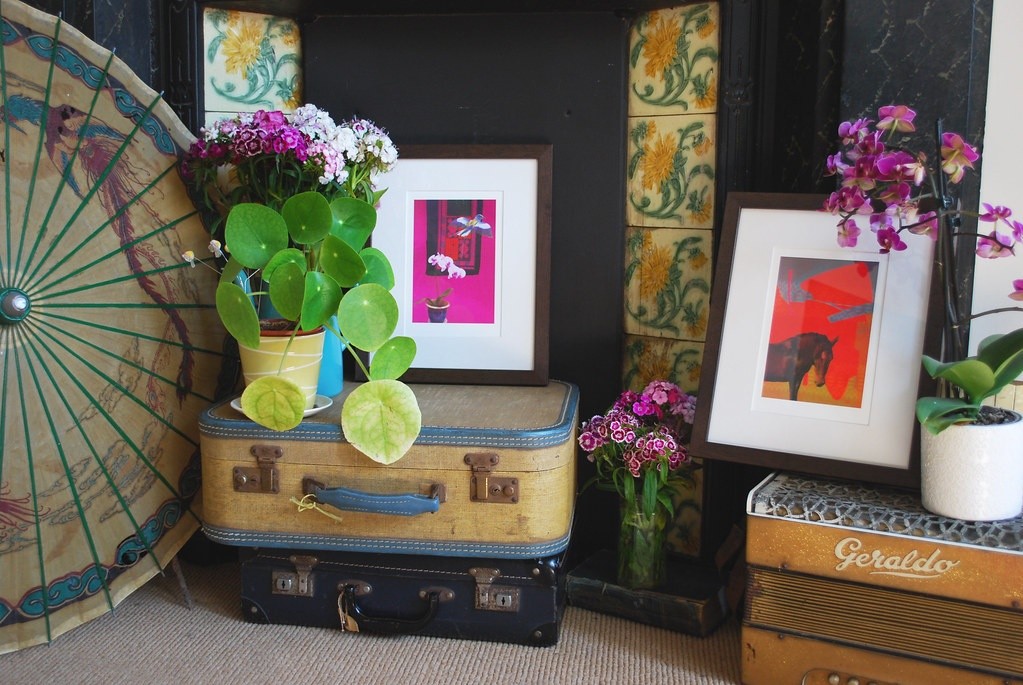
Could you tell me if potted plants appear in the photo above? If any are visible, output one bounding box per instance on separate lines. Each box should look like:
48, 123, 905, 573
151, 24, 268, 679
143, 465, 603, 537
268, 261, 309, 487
215, 191, 422, 465
920, 328, 1023, 521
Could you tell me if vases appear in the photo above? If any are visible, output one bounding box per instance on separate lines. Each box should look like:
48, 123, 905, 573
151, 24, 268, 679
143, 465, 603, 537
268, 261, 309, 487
233, 270, 351, 398
617, 487, 667, 589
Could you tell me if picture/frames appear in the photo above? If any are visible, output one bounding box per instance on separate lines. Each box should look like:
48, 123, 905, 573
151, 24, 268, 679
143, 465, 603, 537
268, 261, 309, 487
354, 143, 553, 386
687, 191, 951, 491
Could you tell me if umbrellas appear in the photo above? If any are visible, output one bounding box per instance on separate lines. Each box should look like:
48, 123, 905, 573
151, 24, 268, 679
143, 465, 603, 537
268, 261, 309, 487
0, 0, 246, 654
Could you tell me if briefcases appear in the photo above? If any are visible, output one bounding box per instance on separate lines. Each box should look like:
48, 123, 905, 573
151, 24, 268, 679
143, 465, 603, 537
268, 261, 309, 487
241, 540, 565, 648
200, 377, 579, 558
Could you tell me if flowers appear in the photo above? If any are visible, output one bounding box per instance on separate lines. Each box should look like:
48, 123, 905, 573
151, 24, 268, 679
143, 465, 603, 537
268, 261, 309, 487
818, 105, 1023, 433
578, 381, 697, 589
181, 103, 399, 274
